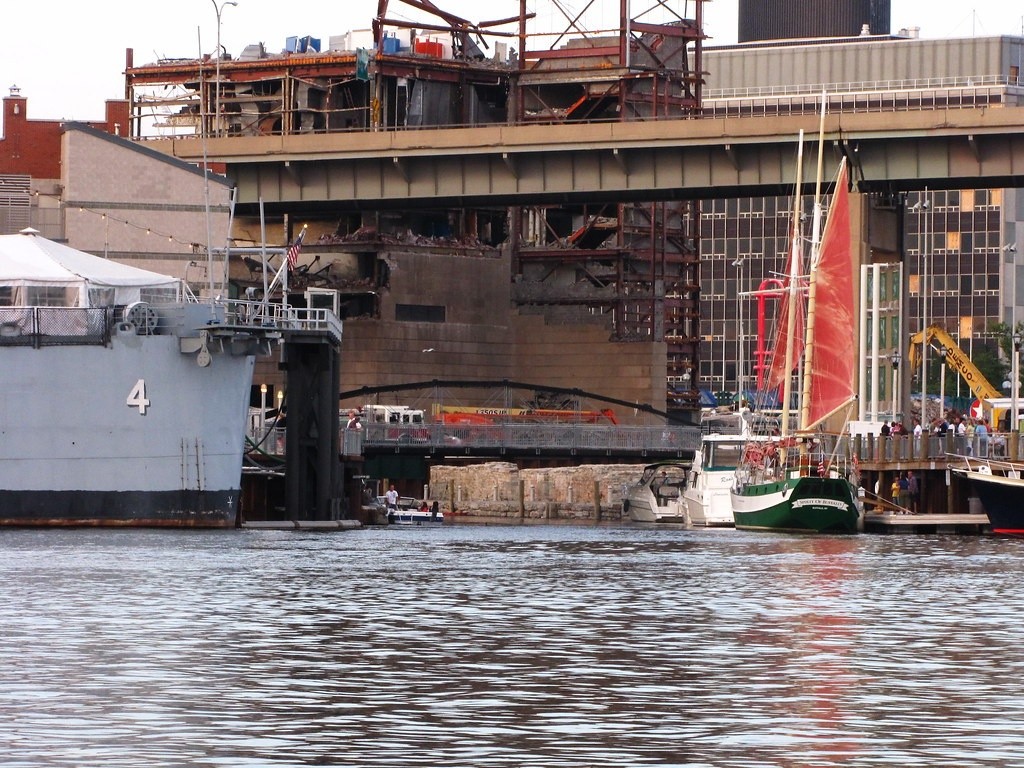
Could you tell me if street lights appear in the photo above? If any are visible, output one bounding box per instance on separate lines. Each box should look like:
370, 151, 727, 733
210, 1, 238, 137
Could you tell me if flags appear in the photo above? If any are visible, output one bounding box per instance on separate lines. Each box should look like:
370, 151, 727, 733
286, 235, 302, 271
817, 459, 825, 473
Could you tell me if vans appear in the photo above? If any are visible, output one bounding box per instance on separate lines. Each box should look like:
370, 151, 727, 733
338, 405, 427, 444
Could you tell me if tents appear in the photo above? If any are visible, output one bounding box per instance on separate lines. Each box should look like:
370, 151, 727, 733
0, 227, 180, 310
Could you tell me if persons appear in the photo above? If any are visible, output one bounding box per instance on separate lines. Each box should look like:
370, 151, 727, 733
385, 485, 399, 524
890, 471, 919, 515
362, 483, 373, 497
662, 471, 672, 483
275, 406, 286, 455
419, 501, 429, 513
346, 412, 363, 430
881, 416, 1010, 461
390, 415, 399, 423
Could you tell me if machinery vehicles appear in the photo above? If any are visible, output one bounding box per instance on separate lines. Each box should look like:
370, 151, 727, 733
432, 403, 622, 446
906, 324, 1024, 436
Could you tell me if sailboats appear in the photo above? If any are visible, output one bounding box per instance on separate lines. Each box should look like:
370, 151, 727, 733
730, 92, 860, 534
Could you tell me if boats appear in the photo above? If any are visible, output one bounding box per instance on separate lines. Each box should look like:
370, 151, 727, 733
944, 452, 1024, 540
681, 434, 865, 530
623, 463, 692, 523
0, 25, 292, 528
370, 496, 444, 524
